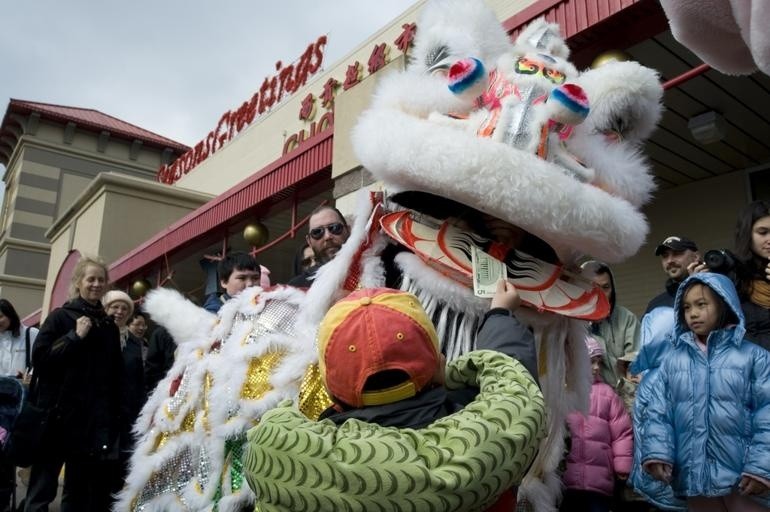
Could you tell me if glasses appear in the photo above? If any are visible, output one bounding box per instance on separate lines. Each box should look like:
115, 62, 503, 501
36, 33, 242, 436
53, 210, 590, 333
308, 222, 348, 240
300, 256, 320, 266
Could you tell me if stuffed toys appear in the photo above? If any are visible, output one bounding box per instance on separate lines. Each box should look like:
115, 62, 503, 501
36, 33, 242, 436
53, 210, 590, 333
110, 0, 665, 511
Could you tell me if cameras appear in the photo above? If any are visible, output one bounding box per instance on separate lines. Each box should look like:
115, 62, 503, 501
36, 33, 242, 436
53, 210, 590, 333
698, 248, 738, 274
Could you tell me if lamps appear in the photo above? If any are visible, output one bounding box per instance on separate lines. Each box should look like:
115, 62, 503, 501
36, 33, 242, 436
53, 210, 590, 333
687, 111, 729, 147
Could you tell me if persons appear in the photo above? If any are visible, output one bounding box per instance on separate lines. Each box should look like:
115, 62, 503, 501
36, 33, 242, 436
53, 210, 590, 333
562, 197, 770, 510
0, 206, 352, 512
270, 277, 542, 509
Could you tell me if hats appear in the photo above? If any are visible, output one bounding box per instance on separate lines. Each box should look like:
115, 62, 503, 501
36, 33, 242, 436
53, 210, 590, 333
319, 286, 442, 407
101, 290, 135, 319
617, 351, 639, 366
585, 336, 603, 358
655, 235, 698, 257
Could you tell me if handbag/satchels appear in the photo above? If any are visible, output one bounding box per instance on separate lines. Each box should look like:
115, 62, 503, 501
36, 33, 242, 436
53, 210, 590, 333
7, 402, 54, 468
